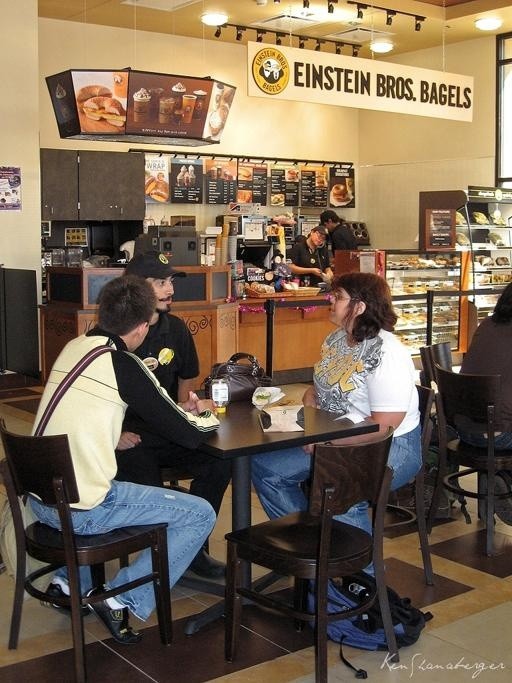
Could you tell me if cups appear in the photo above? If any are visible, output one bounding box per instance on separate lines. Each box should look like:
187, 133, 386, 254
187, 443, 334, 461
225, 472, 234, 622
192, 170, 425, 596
131, 87, 205, 124
216, 221, 238, 264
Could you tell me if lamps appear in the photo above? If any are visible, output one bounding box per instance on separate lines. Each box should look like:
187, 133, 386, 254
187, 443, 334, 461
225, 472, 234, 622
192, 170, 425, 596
211, 22, 361, 59
474, 13, 505, 31
369, 36, 396, 54
199, 9, 230, 27
274, 0, 427, 32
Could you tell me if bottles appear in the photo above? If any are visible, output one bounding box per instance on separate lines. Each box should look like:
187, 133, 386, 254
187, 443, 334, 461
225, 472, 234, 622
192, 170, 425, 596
50, 246, 82, 267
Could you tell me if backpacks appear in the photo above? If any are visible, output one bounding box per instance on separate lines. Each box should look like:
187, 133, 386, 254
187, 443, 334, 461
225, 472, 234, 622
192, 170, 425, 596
304, 567, 433, 654
394, 423, 469, 522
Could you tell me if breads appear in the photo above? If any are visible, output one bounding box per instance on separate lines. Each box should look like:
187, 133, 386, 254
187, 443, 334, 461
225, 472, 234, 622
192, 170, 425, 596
78, 85, 126, 127
332, 183, 348, 202
145, 177, 168, 202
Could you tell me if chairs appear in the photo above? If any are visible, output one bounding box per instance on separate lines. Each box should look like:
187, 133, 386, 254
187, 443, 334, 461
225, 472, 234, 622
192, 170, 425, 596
2, 341, 511, 682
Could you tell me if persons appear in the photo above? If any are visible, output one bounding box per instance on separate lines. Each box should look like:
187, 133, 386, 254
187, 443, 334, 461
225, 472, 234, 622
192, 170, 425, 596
320, 209, 357, 250
453, 282, 512, 526
120, 250, 232, 582
29, 274, 216, 645
251, 271, 424, 576
286, 226, 333, 287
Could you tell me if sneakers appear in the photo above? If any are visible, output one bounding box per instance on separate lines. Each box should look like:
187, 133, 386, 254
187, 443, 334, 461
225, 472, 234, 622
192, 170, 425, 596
35, 579, 90, 619
491, 467, 512, 527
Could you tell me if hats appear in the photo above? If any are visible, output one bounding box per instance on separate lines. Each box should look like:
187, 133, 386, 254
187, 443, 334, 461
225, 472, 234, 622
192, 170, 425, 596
312, 225, 331, 242
319, 208, 340, 227
125, 251, 186, 282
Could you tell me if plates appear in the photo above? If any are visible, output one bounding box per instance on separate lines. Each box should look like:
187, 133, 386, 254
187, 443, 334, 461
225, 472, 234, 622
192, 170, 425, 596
329, 189, 353, 207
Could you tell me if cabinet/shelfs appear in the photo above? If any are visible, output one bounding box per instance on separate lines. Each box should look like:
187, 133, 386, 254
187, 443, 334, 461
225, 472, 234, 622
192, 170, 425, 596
417, 186, 512, 334
79, 149, 145, 222
379, 245, 474, 358
39, 146, 77, 220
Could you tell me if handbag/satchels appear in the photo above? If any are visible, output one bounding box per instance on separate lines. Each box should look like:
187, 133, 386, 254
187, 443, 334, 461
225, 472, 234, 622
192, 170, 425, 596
0, 490, 57, 589
202, 352, 276, 402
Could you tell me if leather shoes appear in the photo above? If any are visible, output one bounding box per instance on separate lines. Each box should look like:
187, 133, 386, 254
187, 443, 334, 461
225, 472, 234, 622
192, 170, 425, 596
196, 548, 227, 579
79, 581, 147, 643
